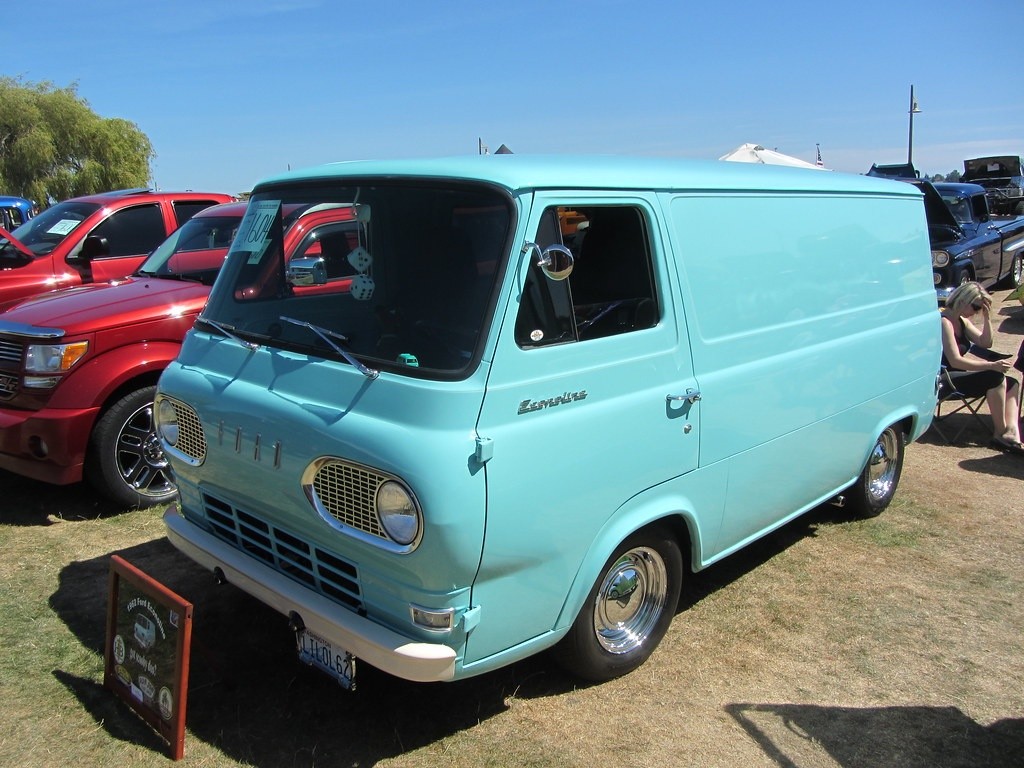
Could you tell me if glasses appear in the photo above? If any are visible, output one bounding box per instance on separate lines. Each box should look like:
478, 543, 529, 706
971, 303, 982, 311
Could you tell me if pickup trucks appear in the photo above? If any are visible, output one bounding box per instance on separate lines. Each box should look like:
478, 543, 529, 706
869, 176, 1024, 302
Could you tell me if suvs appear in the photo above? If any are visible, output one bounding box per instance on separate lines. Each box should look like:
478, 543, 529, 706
0, 201, 364, 508
959, 156, 1024, 216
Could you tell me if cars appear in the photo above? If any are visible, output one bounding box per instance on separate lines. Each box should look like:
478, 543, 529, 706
1, 188, 239, 306
0, 197, 36, 248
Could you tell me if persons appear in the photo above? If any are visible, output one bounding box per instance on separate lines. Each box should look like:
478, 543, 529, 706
938, 281, 1024, 455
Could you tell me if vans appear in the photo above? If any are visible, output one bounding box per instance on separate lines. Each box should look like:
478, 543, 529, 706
151, 156, 943, 691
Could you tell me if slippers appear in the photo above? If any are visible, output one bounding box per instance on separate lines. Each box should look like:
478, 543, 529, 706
989, 434, 1024, 455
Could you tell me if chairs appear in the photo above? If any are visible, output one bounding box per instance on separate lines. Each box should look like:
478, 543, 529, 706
931, 365, 995, 444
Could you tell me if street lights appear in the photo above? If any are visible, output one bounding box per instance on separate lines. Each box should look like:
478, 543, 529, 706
908, 84, 922, 164
